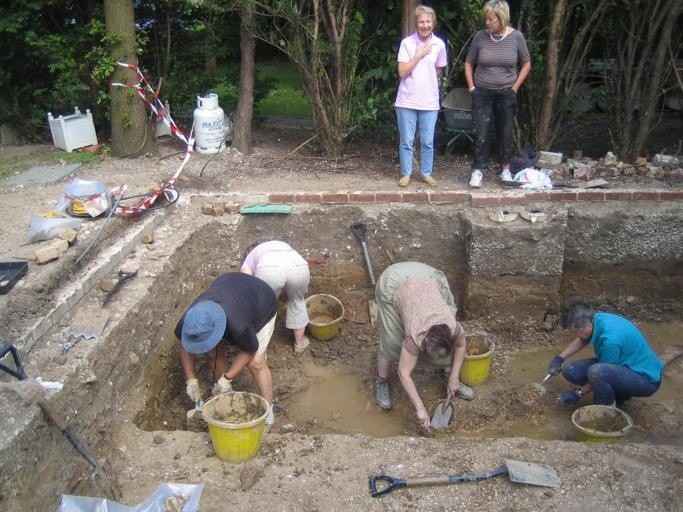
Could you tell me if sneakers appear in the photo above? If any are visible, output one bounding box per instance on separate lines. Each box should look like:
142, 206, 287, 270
468, 171, 483, 188
375, 377, 393, 410
294, 335, 310, 357
444, 374, 475, 400
399, 176, 410, 187
501, 170, 513, 189
421, 176, 437, 187
265, 405, 274, 424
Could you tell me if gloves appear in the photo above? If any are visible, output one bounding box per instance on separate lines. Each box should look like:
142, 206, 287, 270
557, 387, 581, 406
549, 355, 564, 376
212, 374, 233, 396
186, 378, 201, 402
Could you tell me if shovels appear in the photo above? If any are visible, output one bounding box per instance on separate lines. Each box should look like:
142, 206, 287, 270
351, 222, 380, 329
430, 393, 455, 428
515, 373, 554, 407
369, 459, 561, 497
67, 268, 139, 340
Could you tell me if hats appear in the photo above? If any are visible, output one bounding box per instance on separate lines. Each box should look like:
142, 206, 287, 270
181, 299, 227, 354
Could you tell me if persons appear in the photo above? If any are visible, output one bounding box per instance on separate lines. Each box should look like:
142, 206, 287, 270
373, 261, 476, 432
545, 298, 665, 414
464, 0, 532, 190
240, 239, 310, 366
171, 271, 278, 433
390, 5, 449, 188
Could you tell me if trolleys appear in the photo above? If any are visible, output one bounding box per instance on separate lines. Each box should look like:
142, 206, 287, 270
440, 86, 471, 157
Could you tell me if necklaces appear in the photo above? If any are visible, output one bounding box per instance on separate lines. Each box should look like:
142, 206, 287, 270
489, 26, 510, 43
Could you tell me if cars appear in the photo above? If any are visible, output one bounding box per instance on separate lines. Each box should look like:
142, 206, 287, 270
127, 1, 193, 65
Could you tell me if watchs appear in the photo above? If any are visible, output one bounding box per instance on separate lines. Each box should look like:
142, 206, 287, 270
577, 387, 584, 396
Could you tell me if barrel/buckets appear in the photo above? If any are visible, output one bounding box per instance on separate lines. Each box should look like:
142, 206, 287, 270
458, 333, 494, 386
201, 391, 269, 461
303, 293, 345, 340
571, 404, 634, 444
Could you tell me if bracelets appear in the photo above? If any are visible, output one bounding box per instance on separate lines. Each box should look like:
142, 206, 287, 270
468, 87, 475, 93
223, 375, 231, 381
